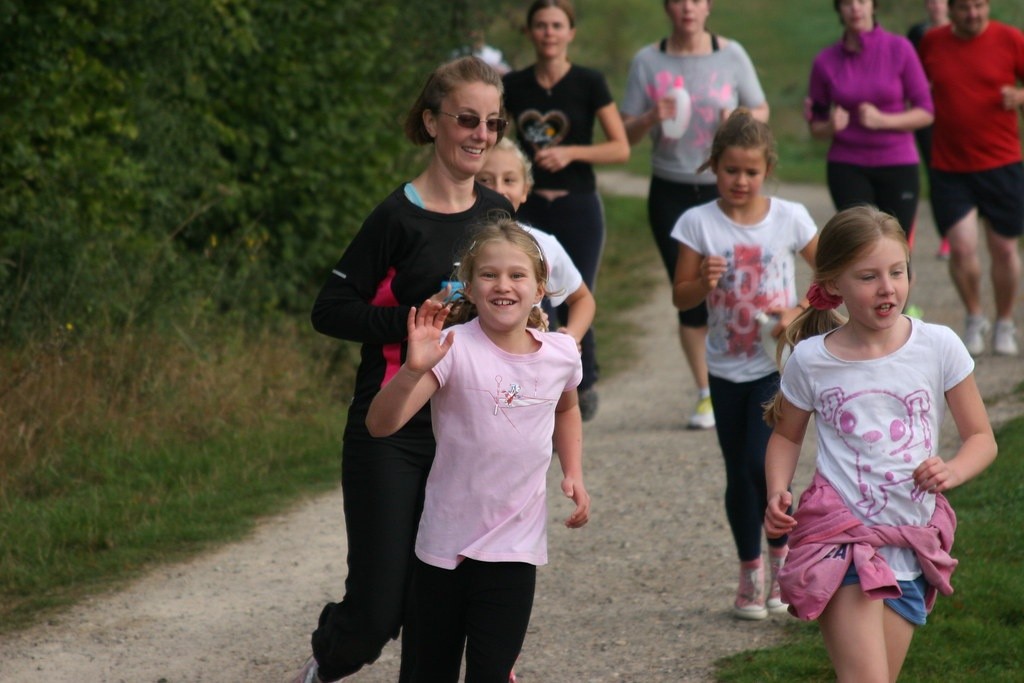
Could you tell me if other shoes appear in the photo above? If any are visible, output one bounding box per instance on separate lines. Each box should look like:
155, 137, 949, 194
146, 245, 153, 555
686, 395, 716, 431
577, 388, 597, 421
990, 319, 1019, 356
961, 316, 991, 358
936, 237, 950, 260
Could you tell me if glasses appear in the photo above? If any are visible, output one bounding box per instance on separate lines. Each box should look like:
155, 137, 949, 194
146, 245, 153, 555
435, 108, 508, 132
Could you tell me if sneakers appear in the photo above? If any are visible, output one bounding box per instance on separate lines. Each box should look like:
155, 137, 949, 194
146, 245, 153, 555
763, 556, 790, 615
288, 654, 345, 683
732, 561, 768, 621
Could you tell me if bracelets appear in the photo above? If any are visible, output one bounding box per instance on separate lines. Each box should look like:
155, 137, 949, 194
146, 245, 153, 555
797, 304, 805, 311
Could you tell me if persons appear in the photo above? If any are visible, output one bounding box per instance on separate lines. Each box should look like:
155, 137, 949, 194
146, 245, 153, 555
920, 0, 1023, 354
622, 0, 770, 430
291, 55, 515, 683
451, 27, 511, 76
764, 206, 998, 683
804, 1, 934, 317
670, 107, 819, 620
366, 221, 591, 683
502, 0, 632, 422
906, 0, 950, 259
476, 136, 595, 353
